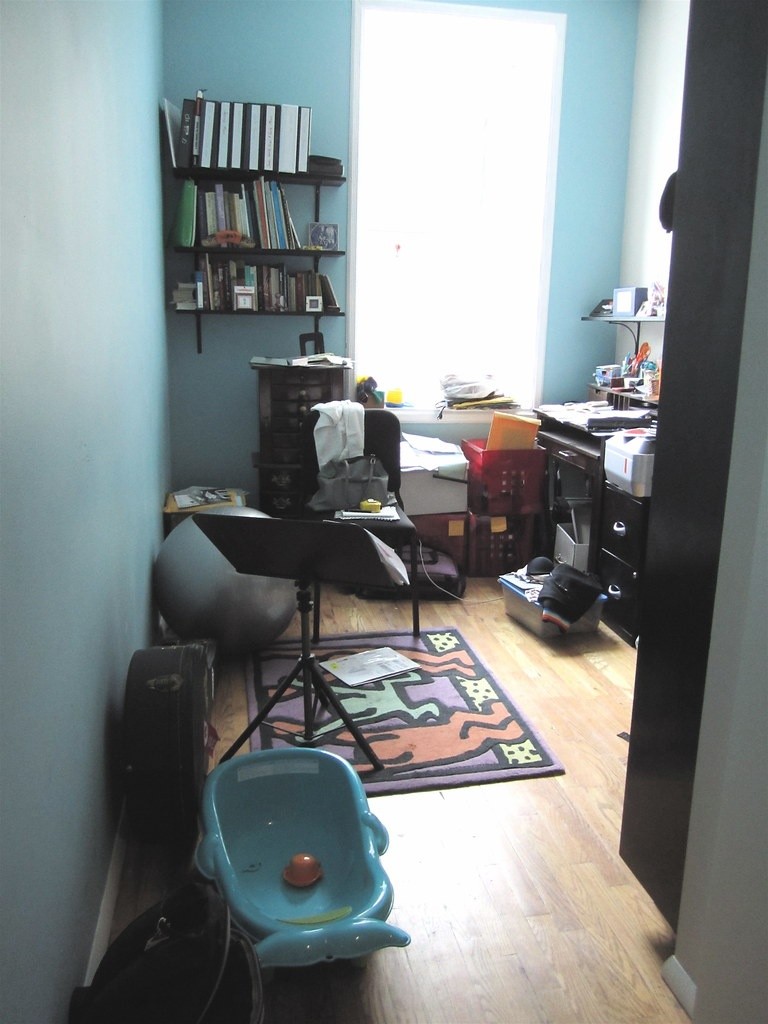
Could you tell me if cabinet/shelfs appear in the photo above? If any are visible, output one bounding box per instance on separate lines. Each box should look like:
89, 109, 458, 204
171, 164, 346, 352
601, 478, 649, 646
250, 359, 348, 515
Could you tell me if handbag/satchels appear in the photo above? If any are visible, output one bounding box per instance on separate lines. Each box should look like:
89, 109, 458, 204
307, 454, 390, 512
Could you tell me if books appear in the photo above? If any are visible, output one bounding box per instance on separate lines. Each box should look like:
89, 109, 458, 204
169, 176, 302, 249
174, 253, 341, 312
162, 90, 312, 174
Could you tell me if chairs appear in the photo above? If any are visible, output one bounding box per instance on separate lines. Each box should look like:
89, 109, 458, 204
299, 409, 420, 643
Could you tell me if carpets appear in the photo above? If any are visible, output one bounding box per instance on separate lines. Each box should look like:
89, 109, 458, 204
245, 625, 565, 799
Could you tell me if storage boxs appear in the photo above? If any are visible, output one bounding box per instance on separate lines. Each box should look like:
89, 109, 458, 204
407, 514, 466, 569
497, 576, 608, 638
555, 523, 591, 572
466, 510, 534, 577
460, 438, 547, 517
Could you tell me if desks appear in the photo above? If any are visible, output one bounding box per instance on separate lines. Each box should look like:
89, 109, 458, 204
533, 407, 652, 575
